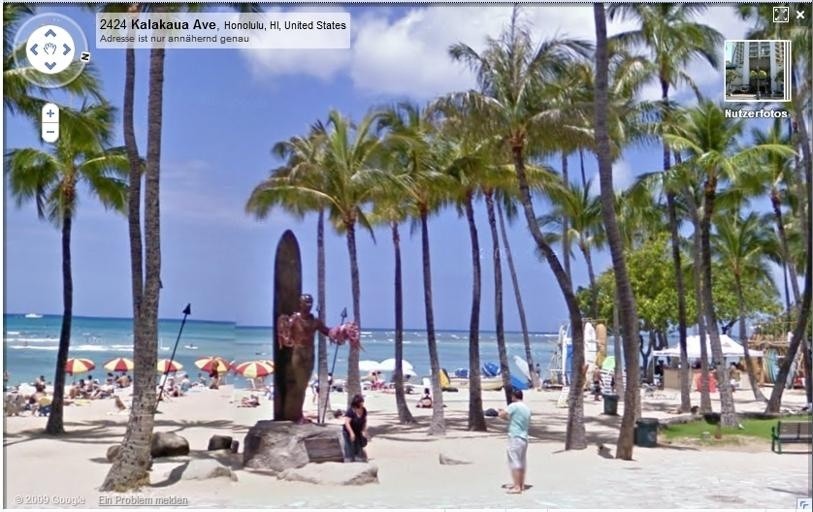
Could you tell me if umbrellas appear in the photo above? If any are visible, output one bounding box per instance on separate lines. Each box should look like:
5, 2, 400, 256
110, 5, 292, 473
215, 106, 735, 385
155, 356, 275, 379
62, 357, 134, 385
358, 358, 417, 376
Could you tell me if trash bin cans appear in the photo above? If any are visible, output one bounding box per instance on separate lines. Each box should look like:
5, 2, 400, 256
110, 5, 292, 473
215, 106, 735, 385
636, 418, 659, 447
603, 393, 620, 414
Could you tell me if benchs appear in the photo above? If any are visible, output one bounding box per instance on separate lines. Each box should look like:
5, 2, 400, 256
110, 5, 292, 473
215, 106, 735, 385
772, 419, 813, 457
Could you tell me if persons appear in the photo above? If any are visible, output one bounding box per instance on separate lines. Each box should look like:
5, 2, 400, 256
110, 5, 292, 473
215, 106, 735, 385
417, 388, 432, 407
278, 294, 356, 424
156, 372, 220, 404
341, 395, 368, 461
654, 360, 664, 390
240, 377, 274, 407
370, 372, 385, 383
3, 372, 132, 419
498, 389, 531, 494
691, 356, 741, 392
535, 363, 541, 378
592, 365, 603, 402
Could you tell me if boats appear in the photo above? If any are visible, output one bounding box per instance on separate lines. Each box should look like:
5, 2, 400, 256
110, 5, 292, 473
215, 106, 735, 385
183, 343, 199, 350
421, 362, 528, 391
25, 312, 42, 319
